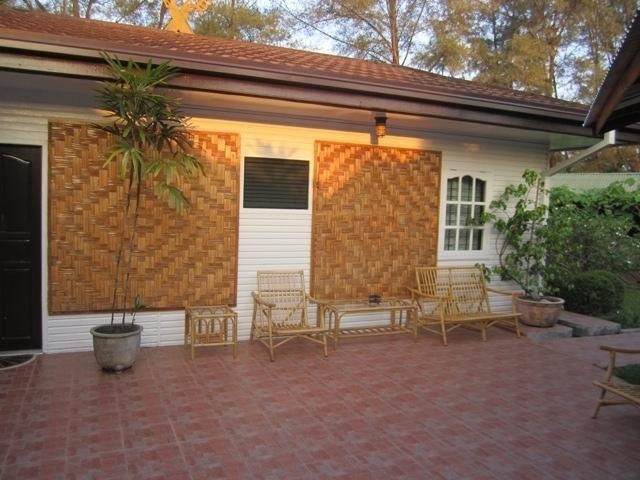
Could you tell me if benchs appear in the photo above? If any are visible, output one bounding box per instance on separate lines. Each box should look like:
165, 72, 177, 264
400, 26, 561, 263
409, 265, 523, 346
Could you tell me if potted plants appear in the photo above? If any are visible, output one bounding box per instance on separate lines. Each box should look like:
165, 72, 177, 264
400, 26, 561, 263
464, 168, 576, 327
89, 50, 206, 372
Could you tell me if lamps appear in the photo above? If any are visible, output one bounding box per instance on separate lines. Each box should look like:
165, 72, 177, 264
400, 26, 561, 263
374, 115, 389, 137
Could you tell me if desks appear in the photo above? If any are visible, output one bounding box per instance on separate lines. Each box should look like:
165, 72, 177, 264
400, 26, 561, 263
318, 294, 420, 350
185, 301, 239, 359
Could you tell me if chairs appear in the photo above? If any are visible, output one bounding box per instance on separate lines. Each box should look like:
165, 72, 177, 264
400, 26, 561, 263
251, 270, 329, 361
